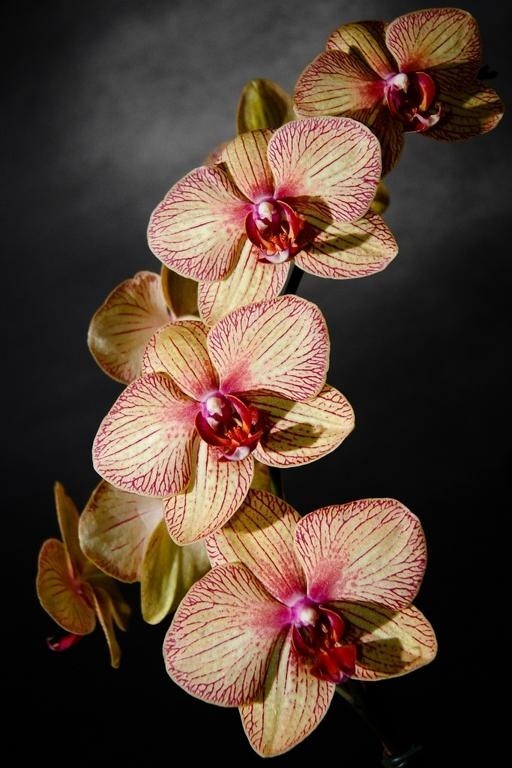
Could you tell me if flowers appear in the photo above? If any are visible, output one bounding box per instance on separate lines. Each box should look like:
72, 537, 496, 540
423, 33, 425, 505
34, 11, 507, 757
